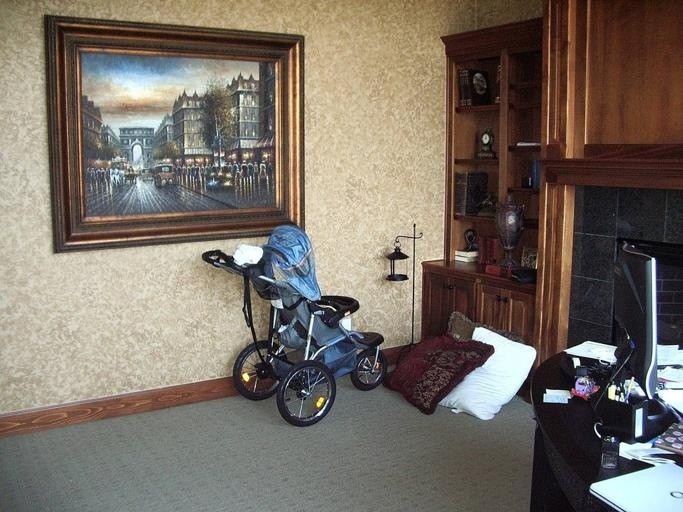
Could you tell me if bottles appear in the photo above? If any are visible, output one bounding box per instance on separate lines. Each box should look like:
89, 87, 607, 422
602, 435, 617, 469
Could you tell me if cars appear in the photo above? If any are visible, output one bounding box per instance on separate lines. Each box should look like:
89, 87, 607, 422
138, 168, 154, 183
153, 163, 180, 188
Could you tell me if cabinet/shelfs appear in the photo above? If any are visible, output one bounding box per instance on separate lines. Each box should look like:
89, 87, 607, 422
422, 17, 543, 338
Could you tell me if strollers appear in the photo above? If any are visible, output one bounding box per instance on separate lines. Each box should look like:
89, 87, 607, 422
199, 219, 388, 429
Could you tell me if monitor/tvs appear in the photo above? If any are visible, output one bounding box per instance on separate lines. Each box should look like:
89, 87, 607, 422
591, 240, 670, 434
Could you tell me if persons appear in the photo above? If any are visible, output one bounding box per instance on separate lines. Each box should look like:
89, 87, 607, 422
174, 159, 272, 188
86, 166, 124, 188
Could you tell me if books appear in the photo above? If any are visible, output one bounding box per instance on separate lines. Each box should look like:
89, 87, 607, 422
458, 70, 489, 106
653, 420, 683, 456
454, 256, 477, 264
452, 170, 489, 218
454, 249, 479, 258
516, 141, 540, 146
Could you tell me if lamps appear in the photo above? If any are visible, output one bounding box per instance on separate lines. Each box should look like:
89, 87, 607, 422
385, 223, 423, 352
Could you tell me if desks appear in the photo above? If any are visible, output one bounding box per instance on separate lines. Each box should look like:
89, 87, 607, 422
529, 348, 682, 510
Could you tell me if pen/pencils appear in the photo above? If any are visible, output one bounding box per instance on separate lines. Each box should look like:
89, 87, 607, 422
615, 376, 635, 404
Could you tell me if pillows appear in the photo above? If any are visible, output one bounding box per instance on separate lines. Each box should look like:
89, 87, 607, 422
381, 310, 537, 421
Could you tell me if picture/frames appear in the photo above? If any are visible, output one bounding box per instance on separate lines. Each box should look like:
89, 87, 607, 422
44, 13, 304, 256
